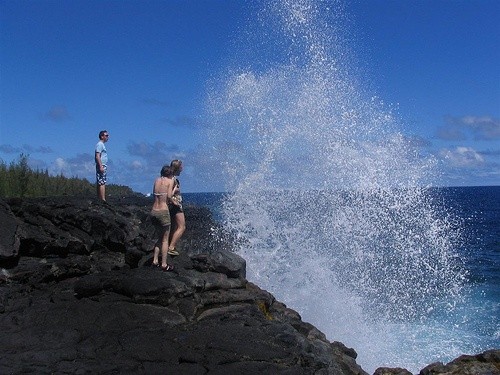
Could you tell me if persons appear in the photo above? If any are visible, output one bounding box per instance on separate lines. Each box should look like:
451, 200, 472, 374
167, 160, 186, 255
151, 166, 180, 271
95, 130, 109, 201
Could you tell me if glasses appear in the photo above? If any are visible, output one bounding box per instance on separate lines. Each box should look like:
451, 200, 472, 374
104, 135, 108, 137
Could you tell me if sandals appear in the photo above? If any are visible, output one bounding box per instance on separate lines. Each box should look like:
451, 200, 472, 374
151, 262, 175, 272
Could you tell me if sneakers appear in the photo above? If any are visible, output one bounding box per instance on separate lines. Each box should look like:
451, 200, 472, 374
168, 248, 180, 256
